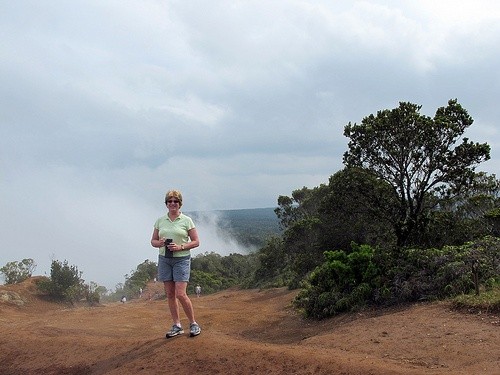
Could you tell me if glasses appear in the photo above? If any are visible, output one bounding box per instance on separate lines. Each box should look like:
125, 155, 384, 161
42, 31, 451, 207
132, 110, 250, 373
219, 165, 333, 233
167, 200, 179, 203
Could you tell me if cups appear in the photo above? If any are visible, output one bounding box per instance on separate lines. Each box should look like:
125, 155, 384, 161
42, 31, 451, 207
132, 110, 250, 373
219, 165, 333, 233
165, 239, 173, 258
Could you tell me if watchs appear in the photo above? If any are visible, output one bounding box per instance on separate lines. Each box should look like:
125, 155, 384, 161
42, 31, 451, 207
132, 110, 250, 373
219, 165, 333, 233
181, 245, 184, 250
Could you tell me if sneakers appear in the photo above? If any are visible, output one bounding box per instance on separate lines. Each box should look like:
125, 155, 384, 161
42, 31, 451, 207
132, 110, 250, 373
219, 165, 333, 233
189, 322, 201, 337
166, 325, 184, 337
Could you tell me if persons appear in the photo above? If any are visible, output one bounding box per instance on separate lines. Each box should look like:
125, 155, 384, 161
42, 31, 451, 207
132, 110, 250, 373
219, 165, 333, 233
151, 190, 201, 338
